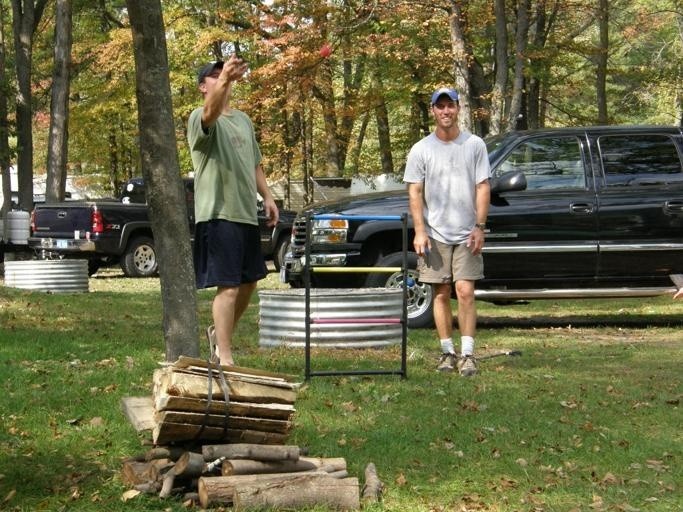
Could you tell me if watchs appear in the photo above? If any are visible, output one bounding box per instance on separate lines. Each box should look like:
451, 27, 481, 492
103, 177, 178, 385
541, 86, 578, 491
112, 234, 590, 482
475, 223, 486, 231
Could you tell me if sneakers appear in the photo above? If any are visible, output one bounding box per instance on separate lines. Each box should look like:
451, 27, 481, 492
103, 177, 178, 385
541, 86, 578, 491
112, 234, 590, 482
458, 355, 477, 376
437, 352, 454, 371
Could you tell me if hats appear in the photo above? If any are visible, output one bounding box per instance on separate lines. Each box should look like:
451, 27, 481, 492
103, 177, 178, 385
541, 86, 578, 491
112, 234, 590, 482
432, 88, 459, 106
198, 61, 224, 82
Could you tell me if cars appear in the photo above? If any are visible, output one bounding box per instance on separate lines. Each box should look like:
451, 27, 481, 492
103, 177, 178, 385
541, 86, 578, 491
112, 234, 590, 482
279, 125, 682, 328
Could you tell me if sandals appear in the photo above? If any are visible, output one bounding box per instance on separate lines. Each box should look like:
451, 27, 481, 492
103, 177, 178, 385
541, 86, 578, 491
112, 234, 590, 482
207, 324, 221, 364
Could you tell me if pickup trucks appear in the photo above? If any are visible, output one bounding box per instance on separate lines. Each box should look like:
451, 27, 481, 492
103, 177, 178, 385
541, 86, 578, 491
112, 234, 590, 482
26, 177, 297, 276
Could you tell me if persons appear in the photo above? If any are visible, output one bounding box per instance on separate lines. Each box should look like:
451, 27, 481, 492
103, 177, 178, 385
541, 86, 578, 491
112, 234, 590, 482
186, 53, 279, 366
402, 87, 493, 374
